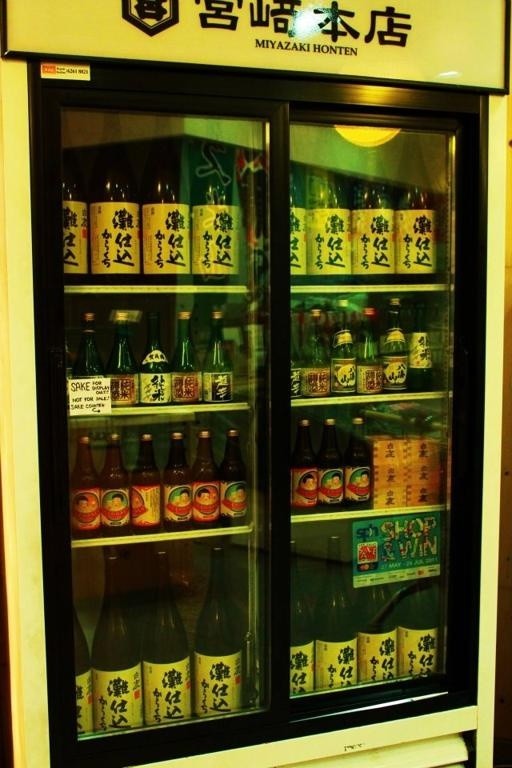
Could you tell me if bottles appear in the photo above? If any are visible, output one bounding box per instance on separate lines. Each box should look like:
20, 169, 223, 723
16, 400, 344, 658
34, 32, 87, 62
53, 154, 456, 740
